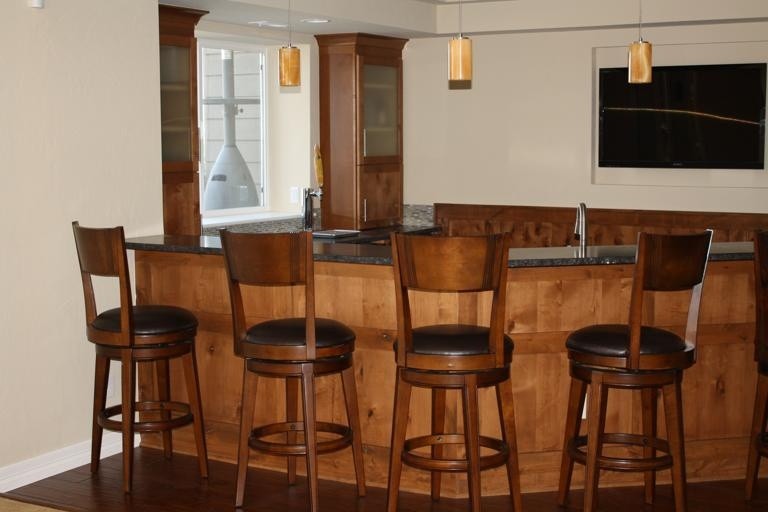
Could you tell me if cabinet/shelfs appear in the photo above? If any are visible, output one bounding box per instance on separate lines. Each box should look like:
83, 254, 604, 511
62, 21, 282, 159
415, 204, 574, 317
316, 39, 409, 229
159, 4, 211, 236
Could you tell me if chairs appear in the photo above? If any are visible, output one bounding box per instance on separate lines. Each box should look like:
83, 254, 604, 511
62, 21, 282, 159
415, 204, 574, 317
742, 228, 766, 511
373, 234, 526, 510
69, 219, 211, 489
553, 230, 715, 508
208, 229, 366, 512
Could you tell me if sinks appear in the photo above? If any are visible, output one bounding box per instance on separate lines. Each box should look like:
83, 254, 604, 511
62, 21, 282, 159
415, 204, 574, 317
311, 228, 362, 238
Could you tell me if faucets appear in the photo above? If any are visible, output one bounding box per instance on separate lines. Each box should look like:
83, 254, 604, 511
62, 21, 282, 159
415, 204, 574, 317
573, 202, 587, 260
301, 186, 323, 232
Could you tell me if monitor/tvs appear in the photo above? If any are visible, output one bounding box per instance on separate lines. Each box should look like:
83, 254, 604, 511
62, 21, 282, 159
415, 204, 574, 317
599, 63, 767, 168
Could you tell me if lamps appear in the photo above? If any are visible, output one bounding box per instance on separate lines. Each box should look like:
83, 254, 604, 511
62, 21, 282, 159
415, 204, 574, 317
445, 1, 476, 90
278, 1, 302, 88
625, 1, 655, 85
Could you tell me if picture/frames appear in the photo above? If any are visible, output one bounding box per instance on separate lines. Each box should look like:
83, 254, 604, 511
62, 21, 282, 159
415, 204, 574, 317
589, 45, 767, 189
